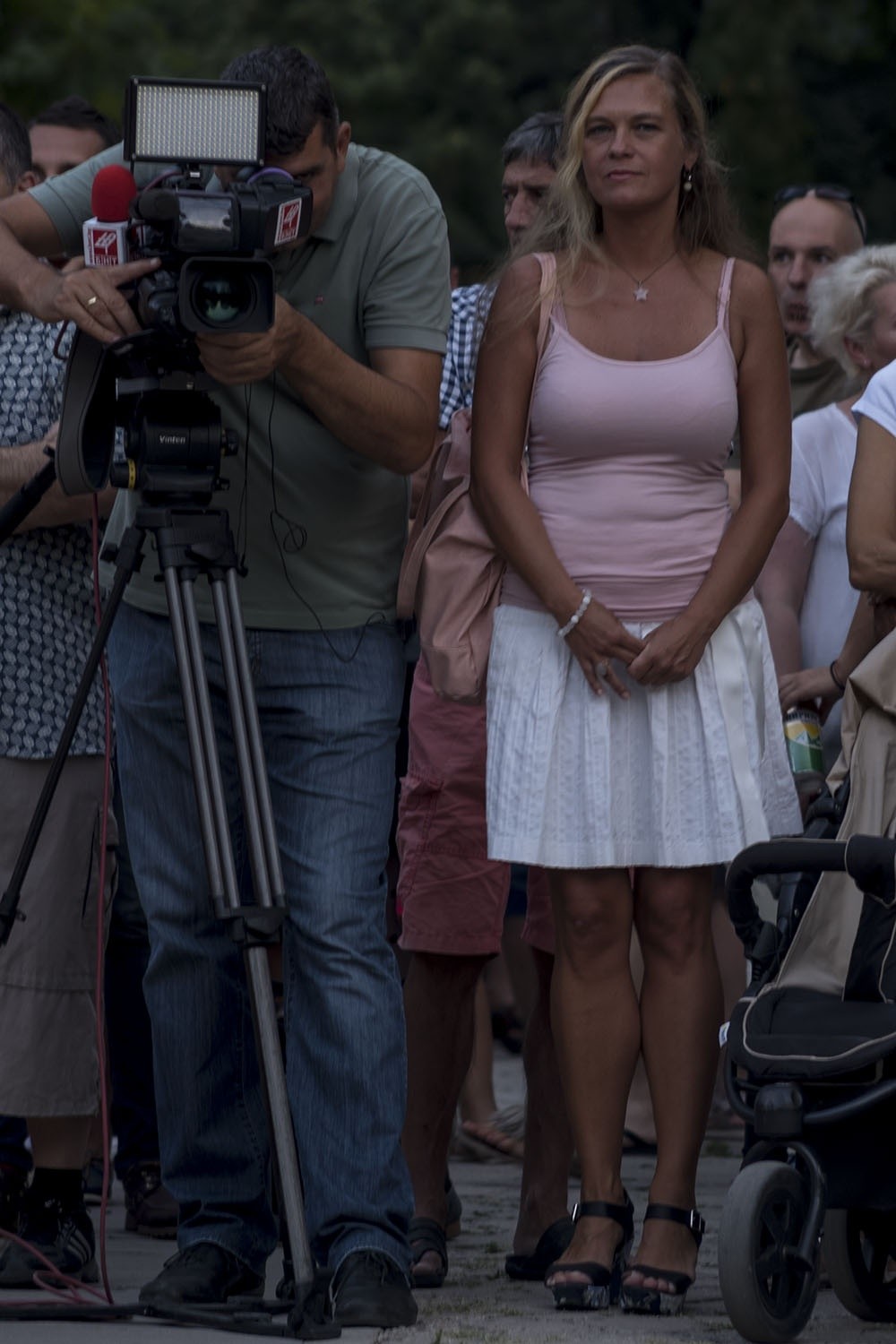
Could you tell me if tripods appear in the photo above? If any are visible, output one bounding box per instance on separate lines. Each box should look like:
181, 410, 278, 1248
0, 365, 340, 1344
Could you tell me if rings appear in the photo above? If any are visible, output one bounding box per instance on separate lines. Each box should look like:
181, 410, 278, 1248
86, 295, 98, 310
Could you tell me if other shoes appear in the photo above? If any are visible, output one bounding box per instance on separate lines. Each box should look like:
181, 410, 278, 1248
333, 1248, 418, 1327
139, 1240, 265, 1309
0, 1194, 100, 1289
621, 1126, 657, 1154
125, 1161, 179, 1236
840, 1276, 896, 1324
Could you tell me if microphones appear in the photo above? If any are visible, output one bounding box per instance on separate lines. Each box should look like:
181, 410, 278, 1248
83, 164, 136, 274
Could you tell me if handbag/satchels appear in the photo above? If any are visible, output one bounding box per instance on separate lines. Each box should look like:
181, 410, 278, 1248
394, 249, 556, 701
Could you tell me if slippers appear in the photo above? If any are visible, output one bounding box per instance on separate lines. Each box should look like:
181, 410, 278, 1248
408, 1216, 448, 1287
506, 1214, 575, 1281
461, 1103, 525, 1160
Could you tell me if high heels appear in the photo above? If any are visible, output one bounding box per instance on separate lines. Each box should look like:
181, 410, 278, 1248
544, 1187, 635, 1312
621, 1201, 708, 1314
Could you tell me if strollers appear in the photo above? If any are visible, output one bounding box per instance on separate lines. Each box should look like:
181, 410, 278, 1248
712, 629, 896, 1344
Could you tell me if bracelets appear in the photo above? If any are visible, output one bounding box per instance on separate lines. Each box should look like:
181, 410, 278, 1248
829, 660, 845, 690
558, 588, 592, 636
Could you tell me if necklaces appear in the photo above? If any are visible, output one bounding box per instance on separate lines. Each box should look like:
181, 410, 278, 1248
612, 245, 683, 303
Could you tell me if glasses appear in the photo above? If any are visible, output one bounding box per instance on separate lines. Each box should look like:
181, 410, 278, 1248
771, 184, 869, 245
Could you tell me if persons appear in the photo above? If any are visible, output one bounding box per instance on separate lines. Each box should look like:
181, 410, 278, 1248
777, 590, 896, 710
0, 52, 450, 1324
469, 49, 802, 1319
30, 108, 744, 1287
708, 186, 866, 518
754, 246, 896, 805
0, 110, 108, 1286
845, 358, 896, 591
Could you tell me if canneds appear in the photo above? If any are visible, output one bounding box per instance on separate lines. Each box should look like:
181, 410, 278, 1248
783, 697, 826, 794
